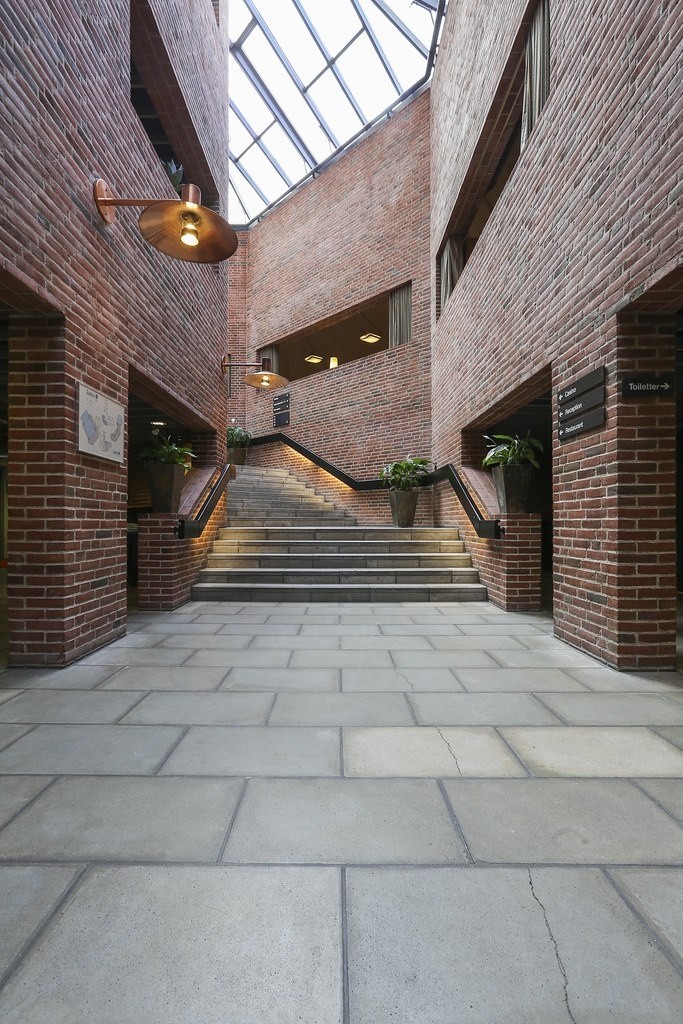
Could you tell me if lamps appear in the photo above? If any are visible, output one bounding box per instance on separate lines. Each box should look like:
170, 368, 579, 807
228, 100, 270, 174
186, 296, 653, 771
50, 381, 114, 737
359, 333, 382, 344
330, 356, 339, 369
305, 354, 323, 363
221, 356, 289, 389
94, 177, 239, 263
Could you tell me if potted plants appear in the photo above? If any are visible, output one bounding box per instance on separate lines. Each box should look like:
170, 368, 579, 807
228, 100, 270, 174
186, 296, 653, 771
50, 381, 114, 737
226, 426, 252, 465
480, 430, 545, 514
139, 433, 197, 514
380, 458, 431, 529
